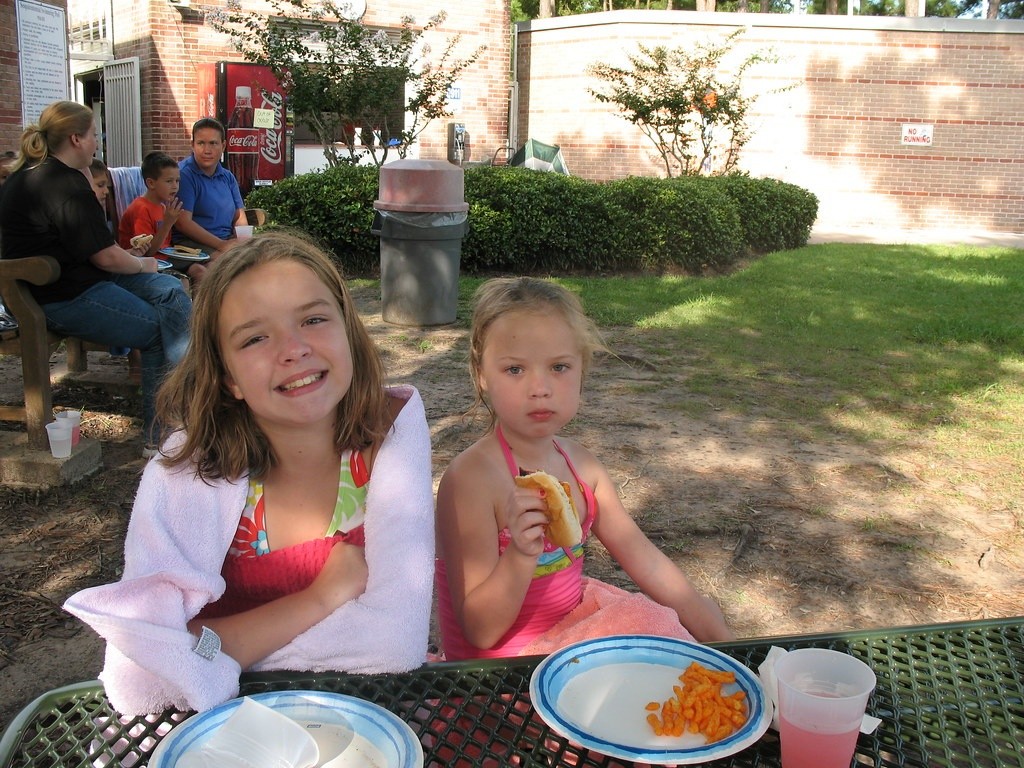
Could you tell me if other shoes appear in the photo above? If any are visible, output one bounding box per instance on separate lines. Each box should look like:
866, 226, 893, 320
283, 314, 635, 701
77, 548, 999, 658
142, 442, 159, 459
0, 304, 19, 330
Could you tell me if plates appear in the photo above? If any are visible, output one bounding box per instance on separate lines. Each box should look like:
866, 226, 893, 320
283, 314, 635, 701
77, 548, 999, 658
136, 257, 173, 270
147, 690, 423, 768
158, 247, 210, 261
530, 634, 773, 764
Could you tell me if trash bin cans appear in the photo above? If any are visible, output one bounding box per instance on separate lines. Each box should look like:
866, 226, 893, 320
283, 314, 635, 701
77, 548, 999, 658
368, 158, 470, 325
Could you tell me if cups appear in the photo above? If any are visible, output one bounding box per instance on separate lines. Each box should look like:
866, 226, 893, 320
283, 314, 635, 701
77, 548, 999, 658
235, 226, 254, 239
46, 423, 72, 457
775, 648, 876, 768
55, 411, 80, 444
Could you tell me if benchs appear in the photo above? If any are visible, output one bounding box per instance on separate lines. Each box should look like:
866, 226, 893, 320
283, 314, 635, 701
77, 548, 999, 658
0, 167, 268, 450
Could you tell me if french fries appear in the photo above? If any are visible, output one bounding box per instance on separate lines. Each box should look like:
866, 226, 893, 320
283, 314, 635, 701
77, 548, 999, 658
645, 662, 747, 746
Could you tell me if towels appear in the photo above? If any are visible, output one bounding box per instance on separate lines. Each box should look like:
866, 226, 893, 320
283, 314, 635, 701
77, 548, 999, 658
517, 576, 697, 656
60, 377, 437, 768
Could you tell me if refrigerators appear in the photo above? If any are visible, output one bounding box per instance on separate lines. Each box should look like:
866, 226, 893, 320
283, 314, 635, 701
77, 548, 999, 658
196, 61, 295, 200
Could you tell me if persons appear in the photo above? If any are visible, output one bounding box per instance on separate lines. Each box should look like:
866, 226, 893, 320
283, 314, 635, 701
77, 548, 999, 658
430, 274, 734, 768
0, 100, 206, 456
61, 228, 434, 768
168, 117, 249, 262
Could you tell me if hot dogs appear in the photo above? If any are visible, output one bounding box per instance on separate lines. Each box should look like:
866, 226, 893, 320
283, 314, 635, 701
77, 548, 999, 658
514, 466, 582, 546
130, 234, 153, 249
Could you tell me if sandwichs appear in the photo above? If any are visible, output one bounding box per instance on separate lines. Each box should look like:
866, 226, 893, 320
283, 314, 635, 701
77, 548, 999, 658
173, 245, 201, 256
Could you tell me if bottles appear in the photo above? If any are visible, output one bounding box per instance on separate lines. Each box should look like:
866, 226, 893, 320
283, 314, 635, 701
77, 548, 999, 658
227, 87, 260, 200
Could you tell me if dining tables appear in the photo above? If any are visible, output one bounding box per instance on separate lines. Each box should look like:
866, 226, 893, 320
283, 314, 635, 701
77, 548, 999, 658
0, 616, 1024, 768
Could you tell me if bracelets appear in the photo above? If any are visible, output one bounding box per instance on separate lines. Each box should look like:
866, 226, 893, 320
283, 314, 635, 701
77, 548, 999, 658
136, 257, 143, 273
